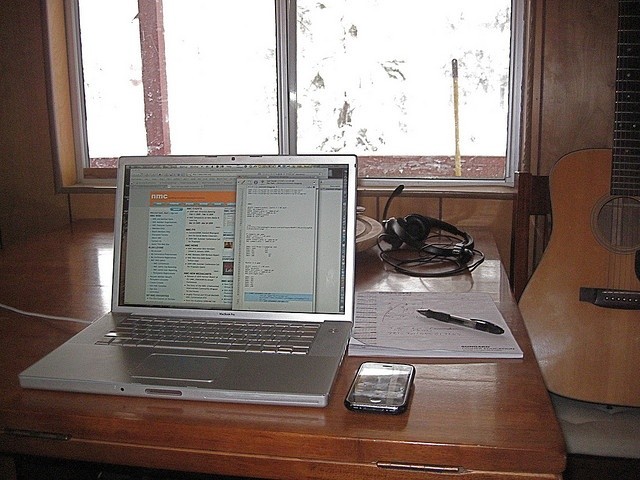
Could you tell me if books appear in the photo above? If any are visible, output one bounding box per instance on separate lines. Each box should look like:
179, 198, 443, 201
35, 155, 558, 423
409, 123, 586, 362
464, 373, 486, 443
348, 290, 523, 360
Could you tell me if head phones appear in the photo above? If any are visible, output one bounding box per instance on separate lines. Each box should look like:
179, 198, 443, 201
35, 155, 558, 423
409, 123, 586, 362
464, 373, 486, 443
382, 214, 474, 257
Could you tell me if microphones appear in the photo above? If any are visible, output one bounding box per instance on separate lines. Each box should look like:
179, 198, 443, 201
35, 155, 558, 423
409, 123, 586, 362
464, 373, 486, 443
382, 185, 404, 223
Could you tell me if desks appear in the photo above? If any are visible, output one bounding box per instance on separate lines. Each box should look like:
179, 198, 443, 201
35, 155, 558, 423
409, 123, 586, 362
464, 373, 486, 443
0, 220, 565, 480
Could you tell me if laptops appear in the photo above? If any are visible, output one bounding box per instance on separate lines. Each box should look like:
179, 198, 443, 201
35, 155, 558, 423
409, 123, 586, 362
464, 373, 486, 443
17, 154, 358, 408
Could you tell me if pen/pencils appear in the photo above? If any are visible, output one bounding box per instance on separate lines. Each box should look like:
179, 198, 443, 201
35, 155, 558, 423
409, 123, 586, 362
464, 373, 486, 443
416, 308, 504, 334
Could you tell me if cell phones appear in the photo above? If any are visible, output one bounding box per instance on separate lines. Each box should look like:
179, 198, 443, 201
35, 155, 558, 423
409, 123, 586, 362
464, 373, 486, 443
344, 361, 415, 415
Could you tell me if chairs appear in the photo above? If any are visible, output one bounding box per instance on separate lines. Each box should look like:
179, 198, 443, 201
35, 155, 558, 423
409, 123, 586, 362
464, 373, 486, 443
510, 172, 640, 479
